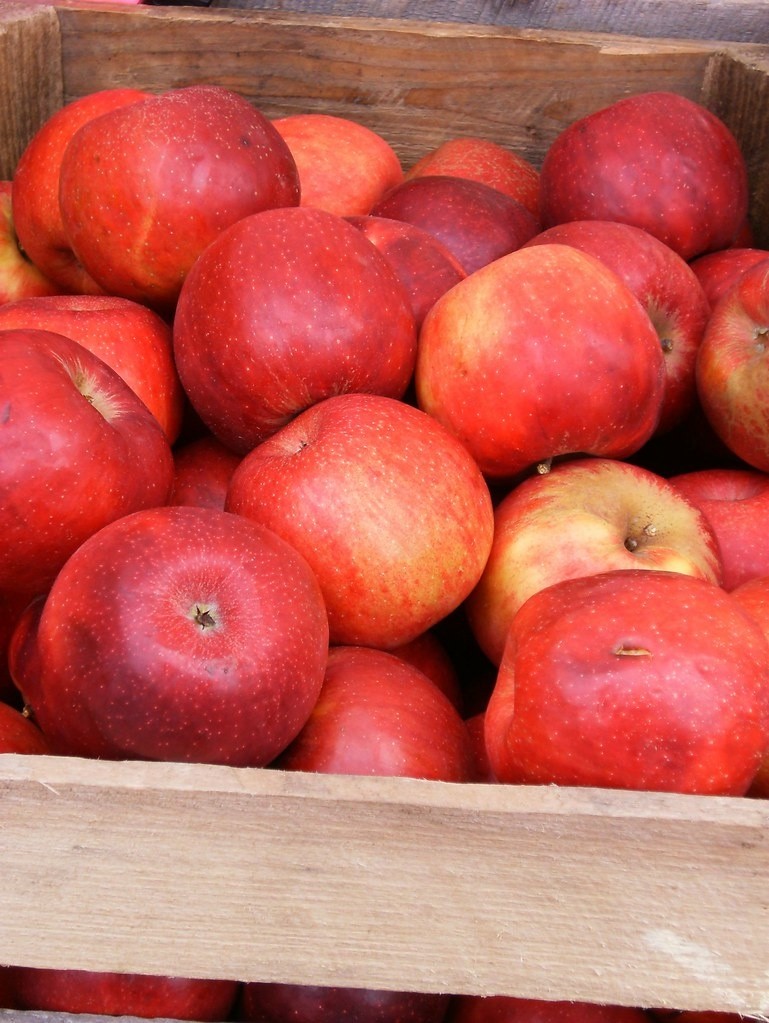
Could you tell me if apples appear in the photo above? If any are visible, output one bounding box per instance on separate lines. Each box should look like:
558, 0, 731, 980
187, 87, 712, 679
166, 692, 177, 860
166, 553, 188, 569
0, 80, 769, 1023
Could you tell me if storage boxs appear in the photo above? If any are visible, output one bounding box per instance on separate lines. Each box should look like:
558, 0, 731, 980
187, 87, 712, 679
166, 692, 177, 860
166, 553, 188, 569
0, 0, 768, 1023
